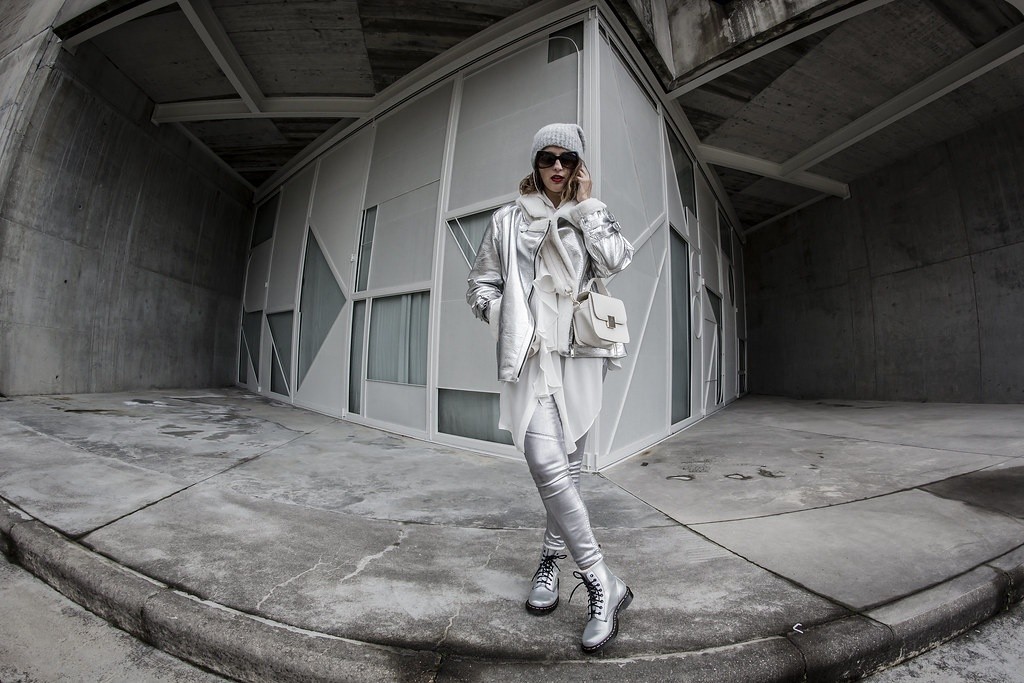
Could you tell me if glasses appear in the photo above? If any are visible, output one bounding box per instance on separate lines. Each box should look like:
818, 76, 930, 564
535, 151, 579, 169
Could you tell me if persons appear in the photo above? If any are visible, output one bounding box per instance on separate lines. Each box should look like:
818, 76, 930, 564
467, 121, 638, 652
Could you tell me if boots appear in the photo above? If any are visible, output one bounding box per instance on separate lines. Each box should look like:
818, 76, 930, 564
524, 543, 562, 615
579, 558, 634, 654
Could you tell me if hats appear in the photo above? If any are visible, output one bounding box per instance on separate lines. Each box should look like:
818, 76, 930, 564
529, 123, 587, 168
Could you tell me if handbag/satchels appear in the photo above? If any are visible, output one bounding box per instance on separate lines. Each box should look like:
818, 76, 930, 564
573, 276, 630, 348
